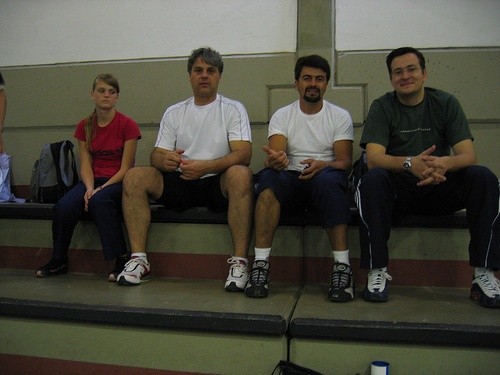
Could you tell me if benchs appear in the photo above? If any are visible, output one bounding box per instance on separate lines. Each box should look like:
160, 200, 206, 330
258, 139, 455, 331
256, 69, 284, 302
0, 202, 500, 375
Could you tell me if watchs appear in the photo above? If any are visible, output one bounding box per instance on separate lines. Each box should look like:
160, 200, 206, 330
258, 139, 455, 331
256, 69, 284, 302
403, 157, 411, 171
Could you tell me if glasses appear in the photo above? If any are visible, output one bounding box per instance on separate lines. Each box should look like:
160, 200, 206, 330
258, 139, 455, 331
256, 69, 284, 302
392, 65, 423, 78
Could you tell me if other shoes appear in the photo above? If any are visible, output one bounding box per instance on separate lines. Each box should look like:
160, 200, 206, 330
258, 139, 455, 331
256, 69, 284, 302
107, 255, 130, 282
35, 262, 69, 278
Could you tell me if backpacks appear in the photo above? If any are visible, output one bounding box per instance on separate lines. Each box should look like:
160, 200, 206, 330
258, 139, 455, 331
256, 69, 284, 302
25, 140, 79, 203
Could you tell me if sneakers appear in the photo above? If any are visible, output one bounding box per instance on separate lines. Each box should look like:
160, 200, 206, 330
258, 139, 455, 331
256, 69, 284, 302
471, 267, 500, 308
116, 253, 151, 286
224, 256, 249, 293
364, 266, 393, 302
328, 261, 354, 302
245, 260, 271, 298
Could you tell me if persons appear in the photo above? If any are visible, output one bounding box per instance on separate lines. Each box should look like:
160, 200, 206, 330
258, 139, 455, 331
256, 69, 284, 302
244, 54, 356, 302
0, 73, 8, 156
352, 47, 500, 308
117, 47, 252, 293
35, 73, 142, 281
